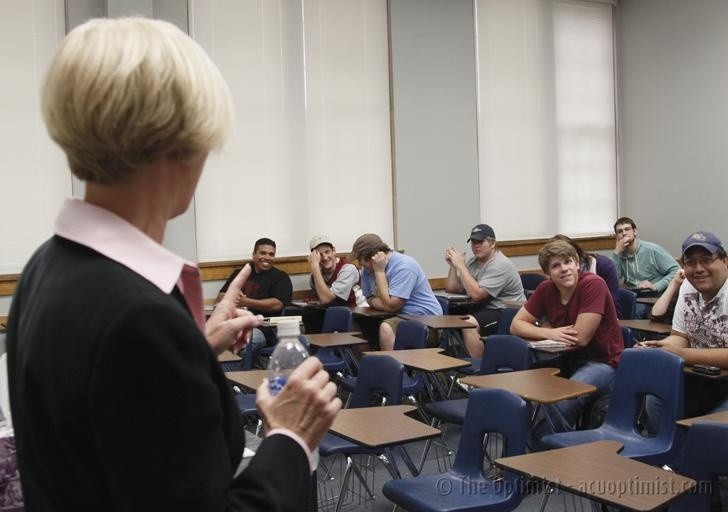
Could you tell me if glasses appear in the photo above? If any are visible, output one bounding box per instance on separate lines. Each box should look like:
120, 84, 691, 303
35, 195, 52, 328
683, 255, 720, 267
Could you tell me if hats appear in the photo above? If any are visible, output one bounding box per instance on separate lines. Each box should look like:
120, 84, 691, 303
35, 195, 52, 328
347, 234, 383, 260
467, 224, 495, 243
682, 230, 721, 254
310, 234, 334, 250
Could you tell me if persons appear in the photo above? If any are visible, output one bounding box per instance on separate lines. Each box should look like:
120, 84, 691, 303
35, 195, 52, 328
444, 224, 528, 359
213, 238, 293, 369
345, 233, 443, 363
6, 16, 345, 512
510, 240, 624, 494
549, 217, 728, 414
308, 234, 368, 358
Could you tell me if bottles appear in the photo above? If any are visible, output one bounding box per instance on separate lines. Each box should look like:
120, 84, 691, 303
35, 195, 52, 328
266, 317, 321, 474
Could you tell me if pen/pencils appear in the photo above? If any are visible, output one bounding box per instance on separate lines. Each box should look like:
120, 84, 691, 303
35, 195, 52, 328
633, 338, 643, 346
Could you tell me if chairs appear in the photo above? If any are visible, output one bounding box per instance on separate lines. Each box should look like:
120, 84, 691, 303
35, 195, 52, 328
203, 274, 728, 512
